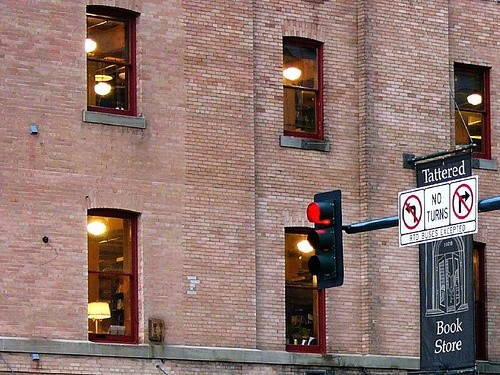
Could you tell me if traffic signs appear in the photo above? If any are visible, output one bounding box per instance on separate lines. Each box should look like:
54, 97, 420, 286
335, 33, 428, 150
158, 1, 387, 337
398, 175, 478, 249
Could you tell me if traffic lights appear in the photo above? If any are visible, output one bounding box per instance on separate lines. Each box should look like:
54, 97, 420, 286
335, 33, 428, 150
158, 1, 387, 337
307, 190, 345, 289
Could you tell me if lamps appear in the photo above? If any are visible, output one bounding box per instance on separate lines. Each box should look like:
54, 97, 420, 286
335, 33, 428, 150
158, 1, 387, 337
86, 294, 115, 339
278, 64, 309, 90
464, 83, 488, 110
87, 217, 111, 239
90, 72, 118, 95
294, 236, 317, 255
